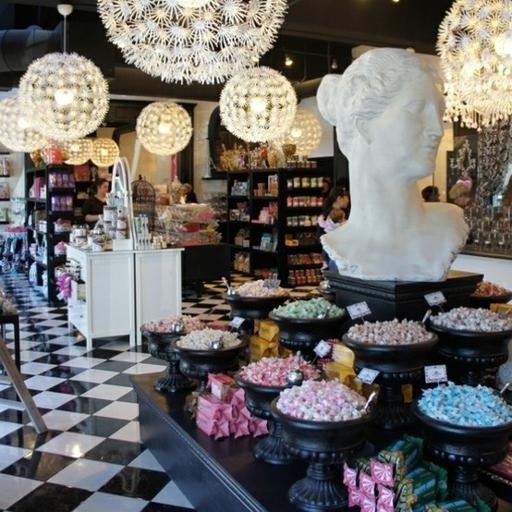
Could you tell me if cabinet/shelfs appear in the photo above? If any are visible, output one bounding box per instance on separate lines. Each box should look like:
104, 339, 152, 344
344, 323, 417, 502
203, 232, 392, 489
224, 156, 334, 285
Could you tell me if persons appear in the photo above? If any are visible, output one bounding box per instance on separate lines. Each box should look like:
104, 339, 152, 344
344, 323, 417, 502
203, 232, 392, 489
448, 182, 473, 245
316, 184, 350, 285
315, 46, 470, 283
421, 185, 442, 203
81, 177, 110, 229
181, 182, 199, 203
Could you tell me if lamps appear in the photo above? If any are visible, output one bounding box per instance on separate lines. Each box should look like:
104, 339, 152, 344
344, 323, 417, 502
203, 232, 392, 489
96, 0, 289, 86
434, 0, 512, 134
